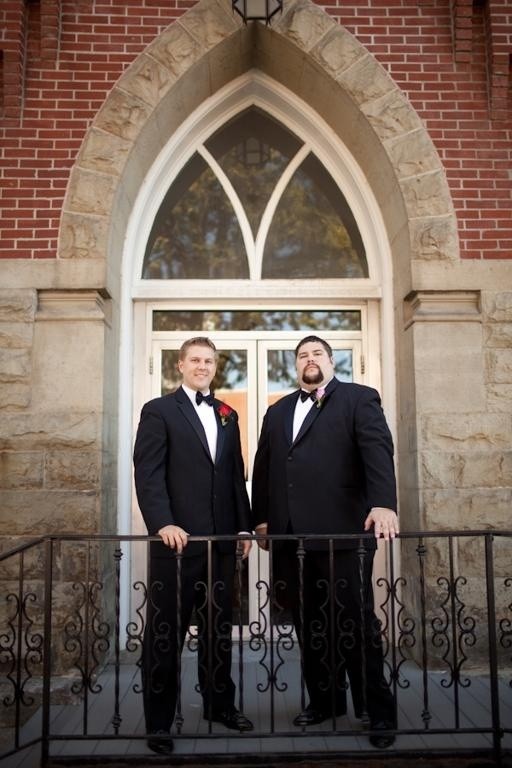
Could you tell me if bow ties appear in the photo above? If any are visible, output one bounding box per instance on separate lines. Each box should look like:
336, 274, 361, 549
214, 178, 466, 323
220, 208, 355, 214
301, 388, 317, 402
196, 391, 215, 406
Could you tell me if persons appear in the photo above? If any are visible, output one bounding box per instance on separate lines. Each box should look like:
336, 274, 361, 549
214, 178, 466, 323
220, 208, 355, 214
249, 336, 400, 747
133, 338, 254, 753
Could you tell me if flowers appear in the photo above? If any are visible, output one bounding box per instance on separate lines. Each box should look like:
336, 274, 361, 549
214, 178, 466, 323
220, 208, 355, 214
216, 404, 233, 427
315, 387, 325, 408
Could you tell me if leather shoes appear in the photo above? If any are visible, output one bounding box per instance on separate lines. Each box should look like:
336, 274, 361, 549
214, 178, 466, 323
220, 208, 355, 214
148, 728, 174, 753
294, 704, 332, 726
215, 709, 253, 730
369, 720, 397, 749
